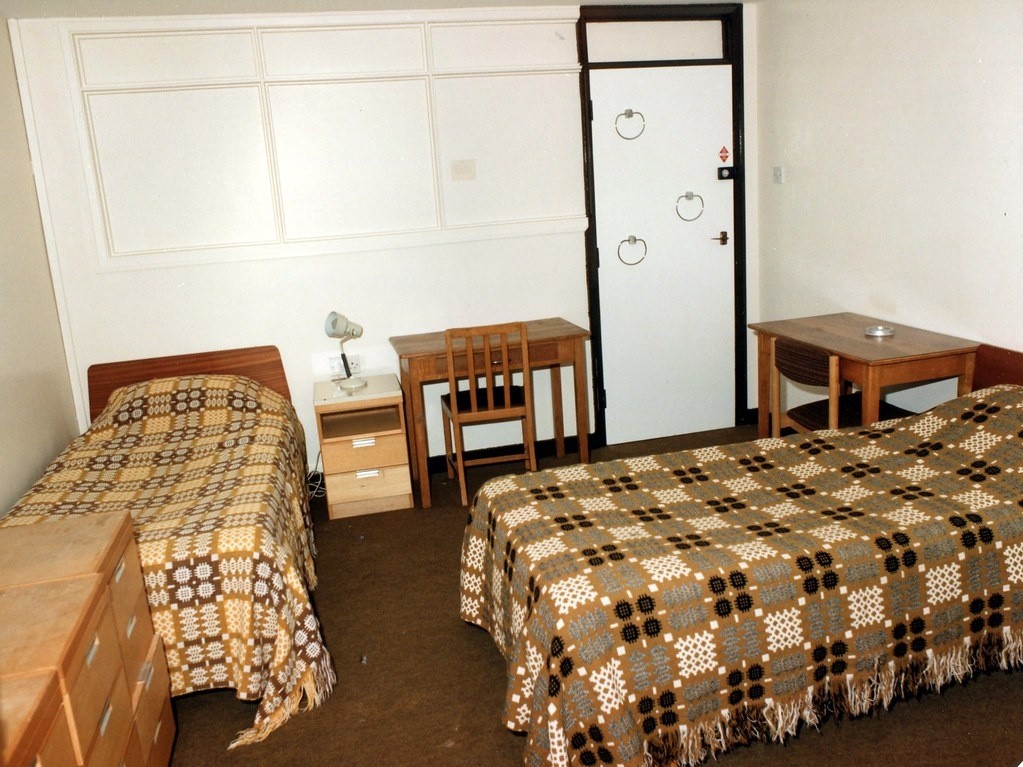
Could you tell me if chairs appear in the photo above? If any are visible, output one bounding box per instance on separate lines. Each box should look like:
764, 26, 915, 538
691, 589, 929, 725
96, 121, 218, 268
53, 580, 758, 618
769, 337, 900, 440
440, 322, 538, 506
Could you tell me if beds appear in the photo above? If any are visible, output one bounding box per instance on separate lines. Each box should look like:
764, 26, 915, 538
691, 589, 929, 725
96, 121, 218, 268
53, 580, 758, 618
0, 345, 337, 752
458, 380, 1023, 767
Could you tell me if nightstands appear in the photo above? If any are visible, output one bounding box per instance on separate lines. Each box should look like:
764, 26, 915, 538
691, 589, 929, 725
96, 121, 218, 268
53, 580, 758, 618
314, 373, 415, 520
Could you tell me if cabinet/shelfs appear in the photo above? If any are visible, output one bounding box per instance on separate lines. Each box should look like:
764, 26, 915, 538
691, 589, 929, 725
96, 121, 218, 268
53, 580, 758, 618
0, 511, 177, 767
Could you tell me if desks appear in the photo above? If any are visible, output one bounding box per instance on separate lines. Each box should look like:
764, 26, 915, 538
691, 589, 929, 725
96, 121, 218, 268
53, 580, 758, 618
748, 312, 979, 440
389, 317, 590, 509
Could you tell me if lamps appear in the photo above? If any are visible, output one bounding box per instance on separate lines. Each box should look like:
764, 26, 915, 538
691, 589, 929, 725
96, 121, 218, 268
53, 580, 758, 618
325, 310, 368, 390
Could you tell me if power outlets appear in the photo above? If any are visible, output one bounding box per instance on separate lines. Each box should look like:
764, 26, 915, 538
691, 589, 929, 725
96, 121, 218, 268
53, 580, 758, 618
346, 354, 360, 373
